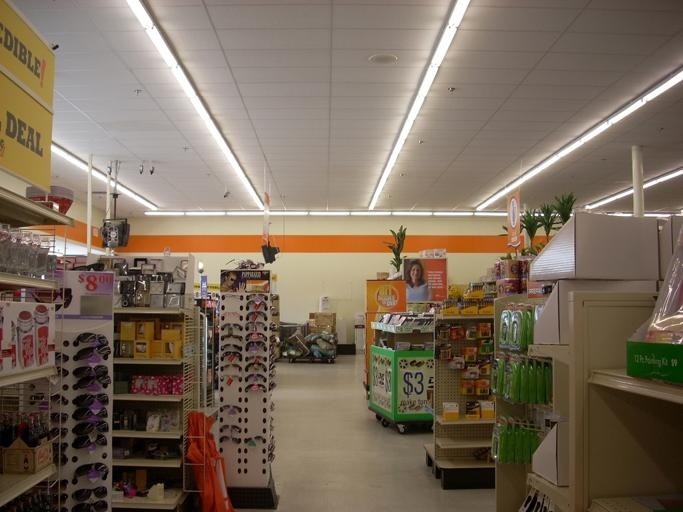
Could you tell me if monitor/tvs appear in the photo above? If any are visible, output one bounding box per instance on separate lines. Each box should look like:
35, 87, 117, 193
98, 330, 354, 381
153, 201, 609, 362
98, 218, 130, 247
262, 244, 280, 264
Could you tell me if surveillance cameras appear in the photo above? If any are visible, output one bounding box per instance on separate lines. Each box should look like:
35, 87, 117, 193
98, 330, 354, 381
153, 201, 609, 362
224, 191, 229, 198
140, 165, 143, 174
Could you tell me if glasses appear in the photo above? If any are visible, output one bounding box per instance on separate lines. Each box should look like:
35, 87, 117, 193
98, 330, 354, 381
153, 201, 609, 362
221, 425, 241, 433
220, 435, 240, 444
221, 323, 242, 378
270, 294, 278, 393
269, 442, 275, 463
245, 294, 267, 393
221, 404, 241, 411
54, 332, 111, 512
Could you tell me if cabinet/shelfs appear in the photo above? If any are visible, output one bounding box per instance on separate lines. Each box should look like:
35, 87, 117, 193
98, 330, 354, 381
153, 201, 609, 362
368, 321, 435, 433
1, 185, 76, 508
57, 255, 214, 511
494, 284, 682, 511
423, 303, 495, 490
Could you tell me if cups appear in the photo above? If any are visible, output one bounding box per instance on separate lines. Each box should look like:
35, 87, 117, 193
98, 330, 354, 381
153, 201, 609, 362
0, 224, 50, 280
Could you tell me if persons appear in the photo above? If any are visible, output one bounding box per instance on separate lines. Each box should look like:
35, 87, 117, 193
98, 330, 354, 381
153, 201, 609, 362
220, 272, 246, 294
406, 260, 431, 301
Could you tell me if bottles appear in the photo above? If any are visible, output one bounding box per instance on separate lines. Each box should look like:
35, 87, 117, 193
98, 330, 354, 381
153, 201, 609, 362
16, 305, 49, 370
0, 410, 50, 448
